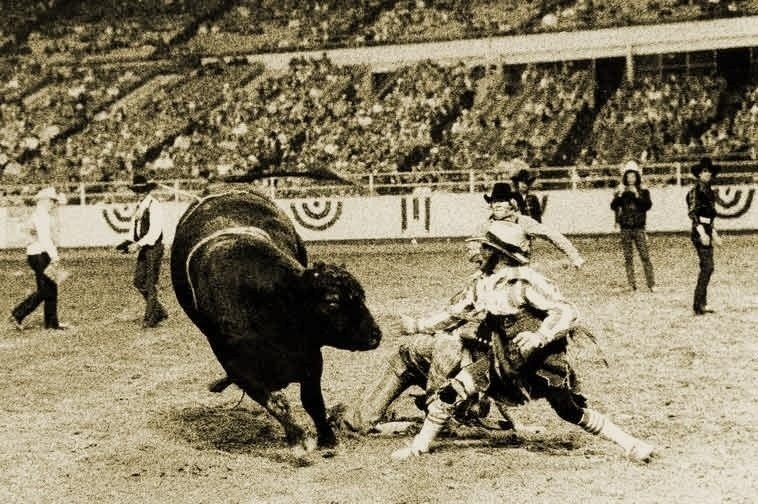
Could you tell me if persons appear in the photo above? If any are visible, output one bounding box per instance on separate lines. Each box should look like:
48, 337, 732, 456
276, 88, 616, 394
6, 184, 71, 334
0, 1, 757, 210
114, 173, 169, 329
510, 169, 541, 222
328, 221, 657, 467
482, 182, 583, 270
682, 156, 723, 315
607, 159, 664, 294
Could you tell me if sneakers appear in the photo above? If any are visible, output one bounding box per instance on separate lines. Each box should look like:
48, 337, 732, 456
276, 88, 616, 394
391, 442, 430, 458
627, 437, 653, 460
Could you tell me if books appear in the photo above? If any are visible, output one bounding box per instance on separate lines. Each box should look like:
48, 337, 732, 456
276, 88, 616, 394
42, 259, 72, 286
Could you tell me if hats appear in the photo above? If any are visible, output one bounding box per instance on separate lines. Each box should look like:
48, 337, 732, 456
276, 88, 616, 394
484, 184, 518, 203
33, 186, 57, 202
511, 169, 537, 185
128, 176, 157, 192
481, 220, 530, 264
691, 158, 721, 178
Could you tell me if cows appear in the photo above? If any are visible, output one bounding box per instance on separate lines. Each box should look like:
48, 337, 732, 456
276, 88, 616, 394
170, 184, 382, 466
